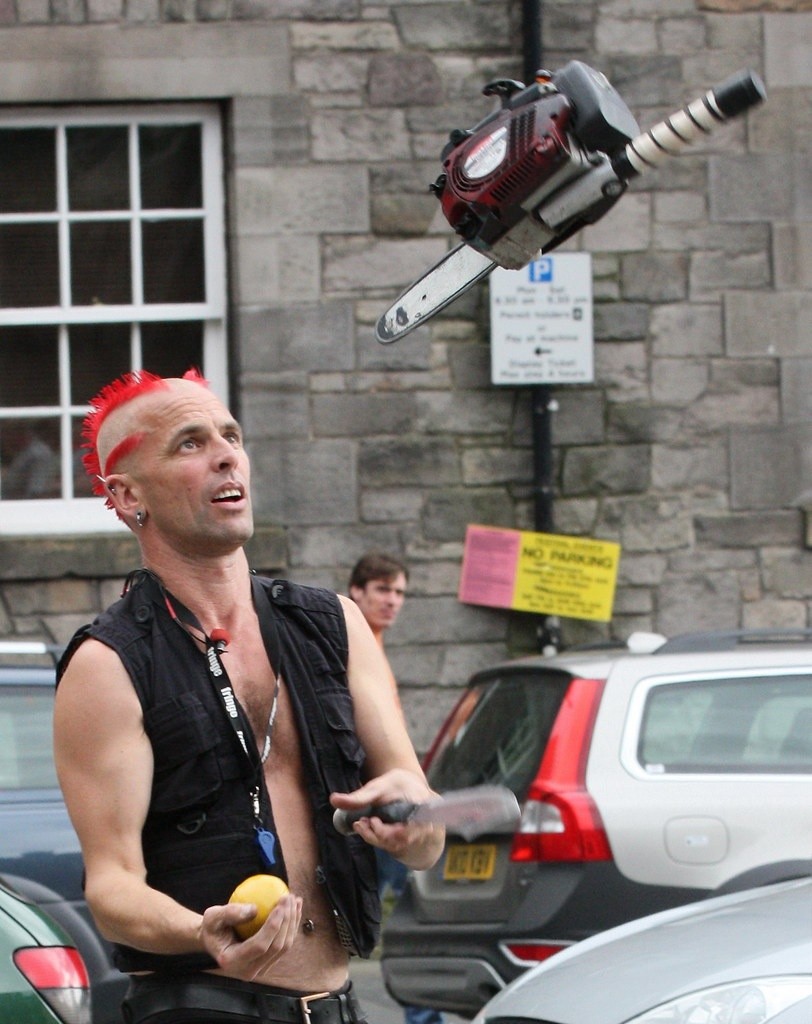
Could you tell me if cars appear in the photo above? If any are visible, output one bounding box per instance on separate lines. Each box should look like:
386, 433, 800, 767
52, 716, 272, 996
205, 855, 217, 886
0, 636, 174, 1009
459, 862, 812, 1024
375, 632, 812, 1015
0, 871, 132, 1024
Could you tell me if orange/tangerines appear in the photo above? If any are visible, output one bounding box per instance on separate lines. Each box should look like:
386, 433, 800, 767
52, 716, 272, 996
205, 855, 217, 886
228, 875, 290, 939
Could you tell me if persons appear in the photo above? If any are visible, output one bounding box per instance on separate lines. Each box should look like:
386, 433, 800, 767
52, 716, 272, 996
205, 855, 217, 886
51, 374, 449, 1023
347, 554, 409, 648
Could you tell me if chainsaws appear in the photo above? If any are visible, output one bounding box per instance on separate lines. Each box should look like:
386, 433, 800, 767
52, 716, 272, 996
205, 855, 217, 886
371, 55, 768, 348
